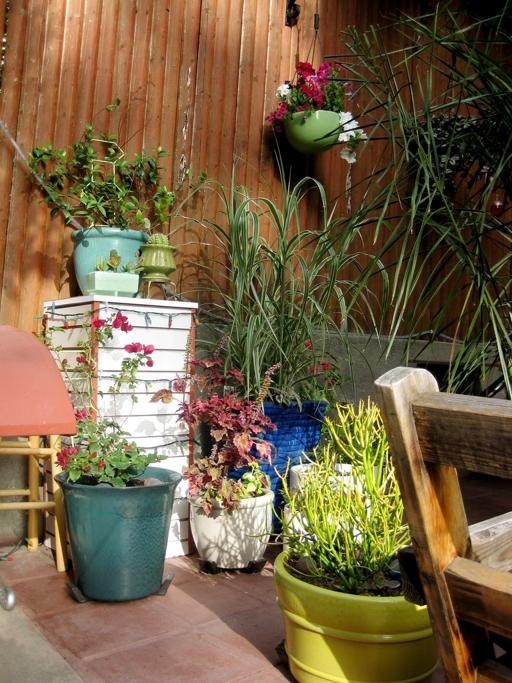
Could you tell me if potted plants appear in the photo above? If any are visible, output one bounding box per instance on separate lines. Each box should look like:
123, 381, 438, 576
14, 97, 213, 295
169, 167, 277, 576
205, 149, 394, 540
272, 395, 446, 683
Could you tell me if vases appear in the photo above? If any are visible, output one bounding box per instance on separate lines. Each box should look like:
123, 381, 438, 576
54, 463, 182, 601
283, 109, 340, 152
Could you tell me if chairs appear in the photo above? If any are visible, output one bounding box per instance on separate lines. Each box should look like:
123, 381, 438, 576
372, 365, 509, 683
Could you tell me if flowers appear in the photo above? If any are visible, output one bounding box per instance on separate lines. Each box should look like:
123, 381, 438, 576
263, 60, 367, 163
32, 306, 169, 488
151, 358, 282, 515
